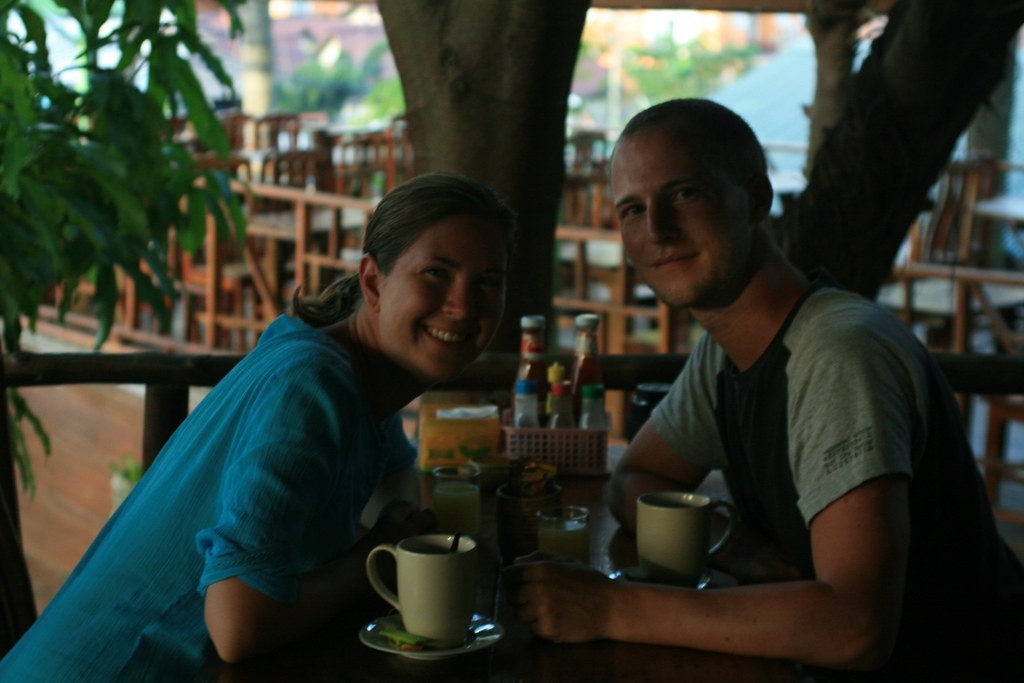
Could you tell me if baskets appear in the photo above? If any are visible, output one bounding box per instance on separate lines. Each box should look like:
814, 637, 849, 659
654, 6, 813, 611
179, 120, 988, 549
503, 427, 611, 475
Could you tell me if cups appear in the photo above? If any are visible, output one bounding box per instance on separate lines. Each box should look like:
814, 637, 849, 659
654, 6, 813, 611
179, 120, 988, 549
365, 534, 479, 651
636, 491, 736, 588
495, 482, 563, 567
430, 464, 481, 538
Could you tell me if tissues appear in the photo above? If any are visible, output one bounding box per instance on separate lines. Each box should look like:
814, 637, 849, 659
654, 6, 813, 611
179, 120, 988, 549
418, 402, 501, 471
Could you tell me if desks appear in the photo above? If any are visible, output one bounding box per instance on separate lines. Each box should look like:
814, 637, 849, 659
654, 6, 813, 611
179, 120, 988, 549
556, 239, 626, 270
217, 385, 807, 683
870, 277, 1024, 351
252, 195, 382, 286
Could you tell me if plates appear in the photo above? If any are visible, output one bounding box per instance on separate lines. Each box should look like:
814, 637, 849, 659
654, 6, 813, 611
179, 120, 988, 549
359, 613, 505, 661
609, 565, 736, 589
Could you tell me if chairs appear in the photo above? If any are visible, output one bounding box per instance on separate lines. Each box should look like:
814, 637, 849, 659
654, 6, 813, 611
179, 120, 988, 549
50, 112, 1024, 542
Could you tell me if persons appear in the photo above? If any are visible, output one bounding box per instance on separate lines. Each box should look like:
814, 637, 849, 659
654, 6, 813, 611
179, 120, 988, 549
1, 172, 516, 683
501, 97, 1024, 683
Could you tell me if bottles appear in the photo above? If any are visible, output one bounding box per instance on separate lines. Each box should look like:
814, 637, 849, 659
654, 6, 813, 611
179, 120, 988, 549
546, 361, 568, 426
514, 315, 547, 400
579, 384, 607, 469
567, 313, 604, 420
546, 384, 577, 467
513, 380, 542, 460
537, 504, 591, 567
